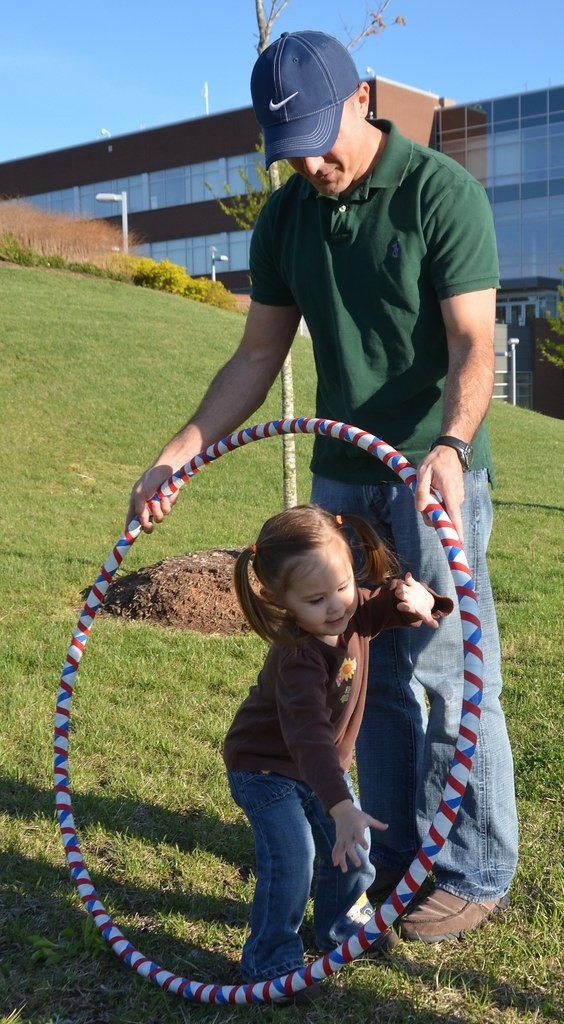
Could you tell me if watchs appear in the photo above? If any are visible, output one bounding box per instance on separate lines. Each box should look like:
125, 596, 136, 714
429, 435, 475, 473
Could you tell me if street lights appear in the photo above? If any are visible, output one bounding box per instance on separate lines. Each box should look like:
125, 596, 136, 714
211, 245, 228, 283
96, 190, 129, 255
494, 338, 519, 406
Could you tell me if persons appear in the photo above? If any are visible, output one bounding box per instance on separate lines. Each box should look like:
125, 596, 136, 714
124, 30, 520, 944
224, 504, 454, 1007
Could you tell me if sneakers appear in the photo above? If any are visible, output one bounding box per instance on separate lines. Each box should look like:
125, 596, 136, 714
357, 929, 399, 961
399, 886, 508, 943
274, 982, 321, 1009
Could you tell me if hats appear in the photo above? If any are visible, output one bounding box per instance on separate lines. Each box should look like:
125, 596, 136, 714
250, 29, 361, 172
